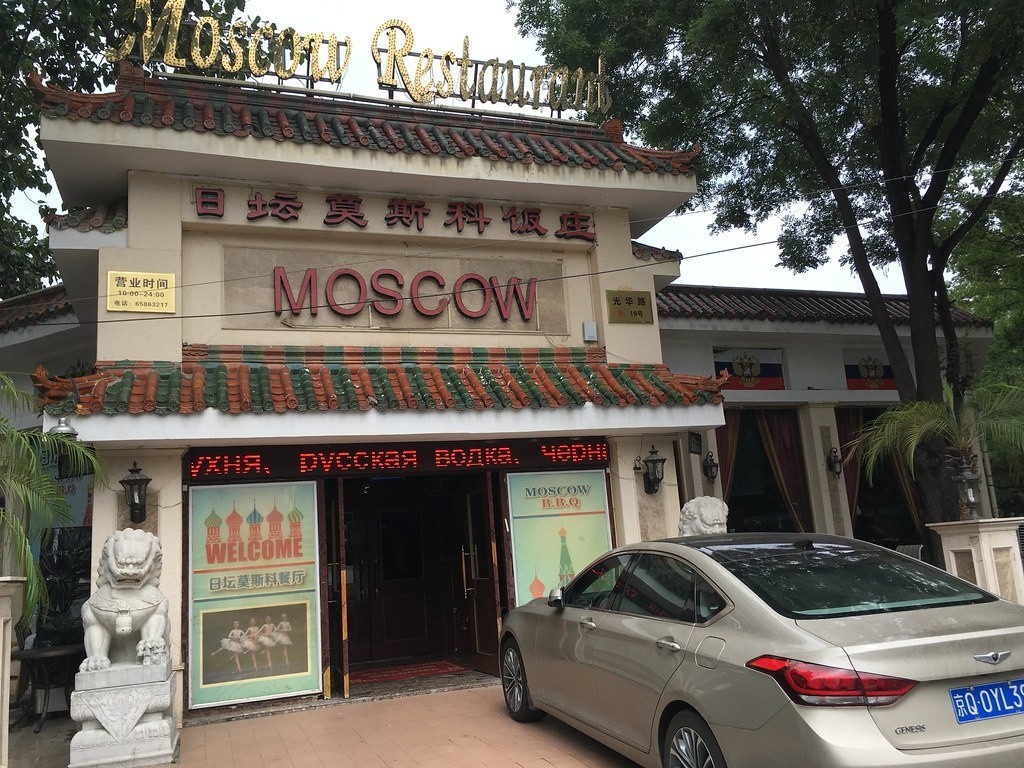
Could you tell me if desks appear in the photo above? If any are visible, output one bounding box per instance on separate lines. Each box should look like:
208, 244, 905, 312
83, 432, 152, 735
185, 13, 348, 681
9, 643, 84, 733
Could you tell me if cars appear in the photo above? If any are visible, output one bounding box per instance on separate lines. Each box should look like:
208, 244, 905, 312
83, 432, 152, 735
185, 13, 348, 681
498, 533, 1024, 768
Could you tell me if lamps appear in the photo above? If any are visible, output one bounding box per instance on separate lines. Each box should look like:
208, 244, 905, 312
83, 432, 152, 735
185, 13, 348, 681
703, 450, 718, 485
633, 444, 667, 494
826, 447, 844, 479
119, 460, 152, 523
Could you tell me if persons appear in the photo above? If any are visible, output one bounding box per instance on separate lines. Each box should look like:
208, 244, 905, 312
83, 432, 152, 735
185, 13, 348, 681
211, 612, 292, 672
